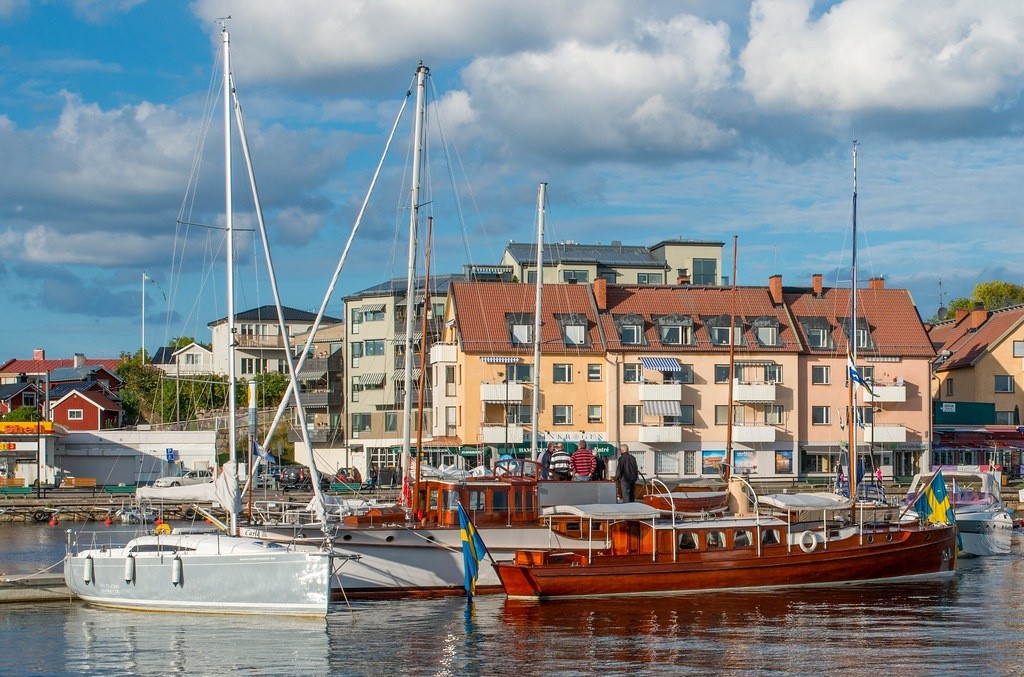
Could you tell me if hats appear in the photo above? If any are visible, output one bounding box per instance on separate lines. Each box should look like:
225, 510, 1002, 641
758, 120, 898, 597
548, 442, 557, 446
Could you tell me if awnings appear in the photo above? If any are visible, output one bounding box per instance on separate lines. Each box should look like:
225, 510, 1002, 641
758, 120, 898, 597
285, 371, 326, 381
459, 446, 492, 457
498, 442, 614, 456
357, 295, 429, 390
640, 358, 682, 372
644, 401, 682, 416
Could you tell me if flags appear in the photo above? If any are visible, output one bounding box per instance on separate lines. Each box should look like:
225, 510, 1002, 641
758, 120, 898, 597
457, 500, 486, 596
915, 470, 964, 557
839, 412, 844, 429
857, 408, 865, 428
253, 440, 275, 463
847, 349, 881, 398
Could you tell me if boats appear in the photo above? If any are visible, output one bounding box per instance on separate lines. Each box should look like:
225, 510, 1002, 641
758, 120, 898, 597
899, 471, 1013, 556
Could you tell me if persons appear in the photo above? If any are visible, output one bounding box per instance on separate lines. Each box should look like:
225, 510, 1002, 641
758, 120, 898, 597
876, 467, 882, 486
721, 459, 727, 478
836, 461, 844, 489
538, 440, 638, 503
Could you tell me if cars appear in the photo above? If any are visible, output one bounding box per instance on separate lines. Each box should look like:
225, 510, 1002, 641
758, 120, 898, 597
154, 469, 213, 487
334, 468, 362, 483
280, 466, 331, 492
259, 466, 286, 481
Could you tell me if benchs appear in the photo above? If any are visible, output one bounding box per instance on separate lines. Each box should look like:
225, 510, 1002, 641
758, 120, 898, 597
59, 477, 96, 490
0, 478, 25, 487
105, 486, 136, 499
895, 475, 921, 487
329, 482, 360, 496
1, 486, 33, 499
806, 477, 833, 488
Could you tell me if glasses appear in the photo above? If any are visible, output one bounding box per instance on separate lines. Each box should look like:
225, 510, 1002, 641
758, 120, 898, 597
619, 449, 623, 451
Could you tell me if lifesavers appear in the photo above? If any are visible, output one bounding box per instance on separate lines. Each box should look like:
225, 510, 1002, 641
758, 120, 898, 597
798, 529, 819, 553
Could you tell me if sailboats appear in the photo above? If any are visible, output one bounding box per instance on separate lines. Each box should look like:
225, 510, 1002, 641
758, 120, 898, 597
490, 138, 964, 601
63, 14, 363, 618
239, 59, 680, 596
